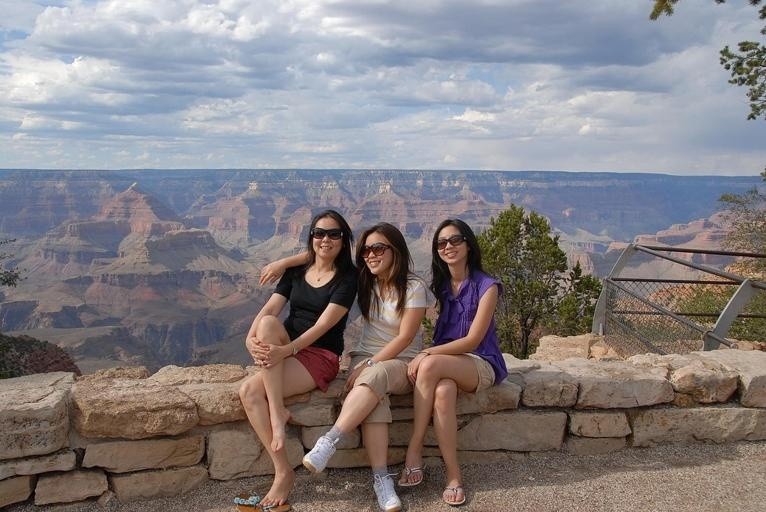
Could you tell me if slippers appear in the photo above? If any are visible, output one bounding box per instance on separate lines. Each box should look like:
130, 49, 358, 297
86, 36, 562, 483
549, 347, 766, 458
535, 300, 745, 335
257, 482, 291, 511
232, 489, 263, 512
397, 460, 427, 488
441, 475, 466, 505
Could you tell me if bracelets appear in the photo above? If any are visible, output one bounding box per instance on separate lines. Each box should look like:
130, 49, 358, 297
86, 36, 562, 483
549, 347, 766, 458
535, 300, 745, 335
361, 357, 374, 367
418, 350, 430, 356
289, 343, 299, 355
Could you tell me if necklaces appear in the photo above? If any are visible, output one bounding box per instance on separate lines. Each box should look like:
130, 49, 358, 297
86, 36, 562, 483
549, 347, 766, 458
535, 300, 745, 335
312, 266, 333, 282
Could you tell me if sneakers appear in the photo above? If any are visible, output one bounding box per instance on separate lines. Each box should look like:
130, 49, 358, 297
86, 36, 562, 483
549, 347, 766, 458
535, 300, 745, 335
301, 436, 340, 475
372, 472, 402, 512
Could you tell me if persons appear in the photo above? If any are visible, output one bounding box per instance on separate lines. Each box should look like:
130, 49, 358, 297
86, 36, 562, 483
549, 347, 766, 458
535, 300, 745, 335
238, 209, 359, 509
302, 222, 427, 512
397, 219, 508, 505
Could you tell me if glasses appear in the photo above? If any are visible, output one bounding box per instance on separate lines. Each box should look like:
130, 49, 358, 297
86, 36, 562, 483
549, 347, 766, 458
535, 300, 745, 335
434, 235, 467, 250
310, 227, 345, 240
359, 242, 393, 258
